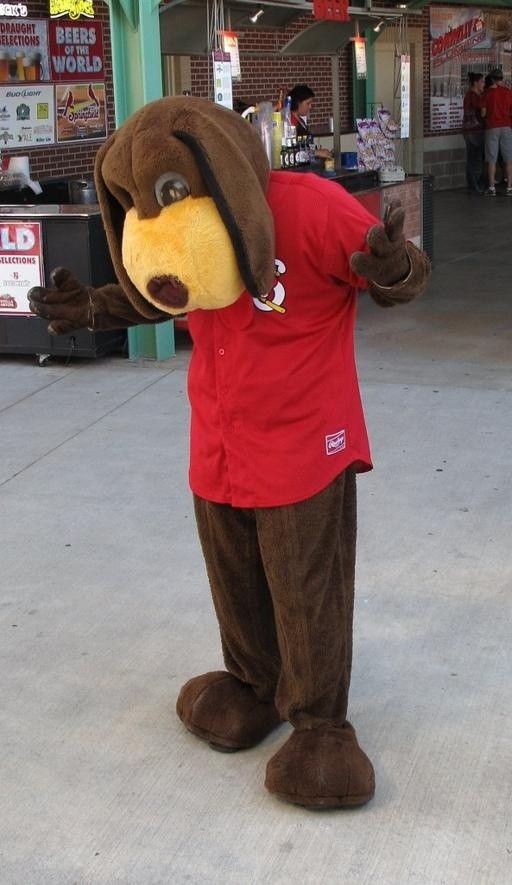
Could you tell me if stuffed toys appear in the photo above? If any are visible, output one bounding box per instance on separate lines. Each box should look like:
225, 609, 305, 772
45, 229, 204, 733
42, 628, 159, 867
26, 94, 433, 812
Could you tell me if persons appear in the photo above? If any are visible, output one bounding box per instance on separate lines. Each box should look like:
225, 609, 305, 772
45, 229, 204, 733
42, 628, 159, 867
478, 71, 501, 185
460, 70, 489, 197
283, 83, 337, 163
477, 67, 512, 197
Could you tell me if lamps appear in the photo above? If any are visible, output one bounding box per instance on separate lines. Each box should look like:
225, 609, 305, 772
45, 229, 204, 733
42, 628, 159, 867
373, 19, 386, 33
248, 1, 265, 23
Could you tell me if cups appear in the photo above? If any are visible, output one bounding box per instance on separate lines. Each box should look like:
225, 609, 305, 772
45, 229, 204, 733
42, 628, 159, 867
0, 50, 43, 81
323, 158, 335, 173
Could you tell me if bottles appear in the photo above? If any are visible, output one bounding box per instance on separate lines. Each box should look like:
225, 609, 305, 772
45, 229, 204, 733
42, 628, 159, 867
282, 95, 292, 136
279, 135, 311, 169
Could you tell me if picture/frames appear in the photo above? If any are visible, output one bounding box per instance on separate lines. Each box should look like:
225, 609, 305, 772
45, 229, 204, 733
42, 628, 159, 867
48, 19, 106, 82
0, 16, 52, 83
55, 83, 109, 145
0, 83, 56, 151
